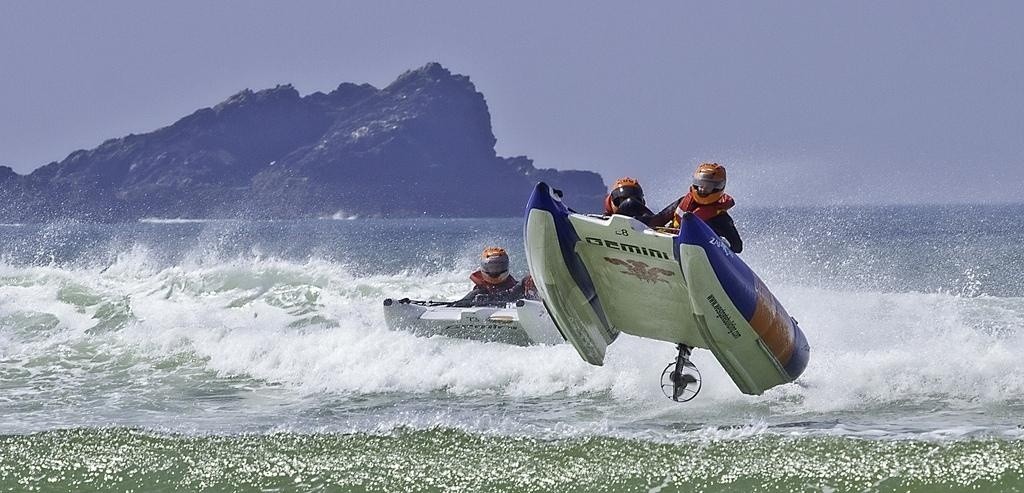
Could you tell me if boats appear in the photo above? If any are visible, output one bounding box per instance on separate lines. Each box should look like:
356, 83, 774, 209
522, 180, 812, 405
383, 296, 566, 348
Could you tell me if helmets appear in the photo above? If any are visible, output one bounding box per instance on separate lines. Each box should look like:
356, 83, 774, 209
689, 163, 725, 203
480, 248, 509, 283
611, 175, 645, 213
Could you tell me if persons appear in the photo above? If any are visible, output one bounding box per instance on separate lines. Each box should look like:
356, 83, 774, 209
643, 161, 744, 254
455, 245, 519, 305
590, 177, 651, 225
